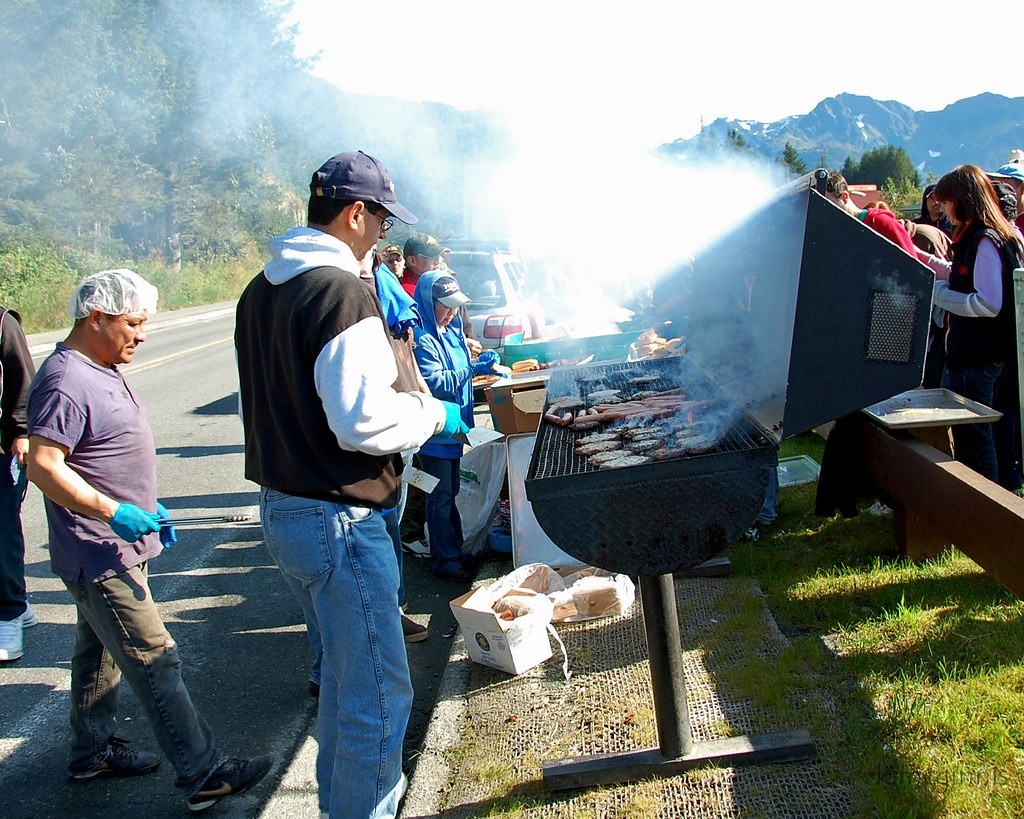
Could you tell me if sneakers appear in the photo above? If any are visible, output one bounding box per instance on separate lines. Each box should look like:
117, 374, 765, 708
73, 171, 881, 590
402, 540, 433, 558
188, 755, 274, 811
68, 735, 162, 780
0, 605, 39, 661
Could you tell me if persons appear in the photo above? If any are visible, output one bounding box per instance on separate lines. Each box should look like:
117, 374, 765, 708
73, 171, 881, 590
0, 306, 39, 662
235, 149, 515, 819
814, 149, 1024, 496
274, 234, 508, 696
26, 269, 273, 812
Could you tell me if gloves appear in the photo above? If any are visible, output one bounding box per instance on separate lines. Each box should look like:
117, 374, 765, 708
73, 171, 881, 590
110, 504, 177, 549
440, 401, 469, 442
472, 353, 508, 379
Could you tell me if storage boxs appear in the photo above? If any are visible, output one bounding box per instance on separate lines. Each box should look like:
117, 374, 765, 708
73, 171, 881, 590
448, 584, 556, 676
573, 584, 628, 616
483, 386, 547, 434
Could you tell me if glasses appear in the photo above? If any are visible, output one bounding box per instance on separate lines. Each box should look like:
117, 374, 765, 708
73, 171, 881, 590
387, 258, 402, 264
374, 212, 394, 232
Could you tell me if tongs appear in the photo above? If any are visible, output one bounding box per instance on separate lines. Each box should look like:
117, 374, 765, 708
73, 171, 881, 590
153, 514, 252, 526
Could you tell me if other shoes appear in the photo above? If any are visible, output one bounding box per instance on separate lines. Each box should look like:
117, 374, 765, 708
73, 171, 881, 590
402, 618, 429, 642
441, 552, 475, 581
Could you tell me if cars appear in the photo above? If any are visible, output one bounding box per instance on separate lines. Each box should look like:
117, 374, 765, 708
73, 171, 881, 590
440, 234, 543, 342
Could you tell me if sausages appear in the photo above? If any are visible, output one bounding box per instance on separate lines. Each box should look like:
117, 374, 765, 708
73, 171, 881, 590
545, 387, 720, 423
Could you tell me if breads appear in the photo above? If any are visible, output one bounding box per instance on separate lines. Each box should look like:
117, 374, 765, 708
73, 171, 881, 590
634, 330, 688, 359
473, 359, 538, 382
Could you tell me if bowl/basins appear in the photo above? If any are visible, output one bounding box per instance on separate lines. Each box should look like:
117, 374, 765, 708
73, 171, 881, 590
488, 526, 513, 552
501, 513, 510, 530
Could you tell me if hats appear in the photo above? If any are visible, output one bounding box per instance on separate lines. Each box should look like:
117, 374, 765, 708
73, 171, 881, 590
927, 186, 936, 197
383, 245, 402, 257
309, 150, 415, 225
986, 163, 1024, 182
403, 233, 452, 258
432, 277, 471, 308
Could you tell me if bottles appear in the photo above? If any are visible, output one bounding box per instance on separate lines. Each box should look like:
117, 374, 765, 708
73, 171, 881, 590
528, 308, 547, 339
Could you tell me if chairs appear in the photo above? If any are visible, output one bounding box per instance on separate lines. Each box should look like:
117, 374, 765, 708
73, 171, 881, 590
468, 280, 496, 299
